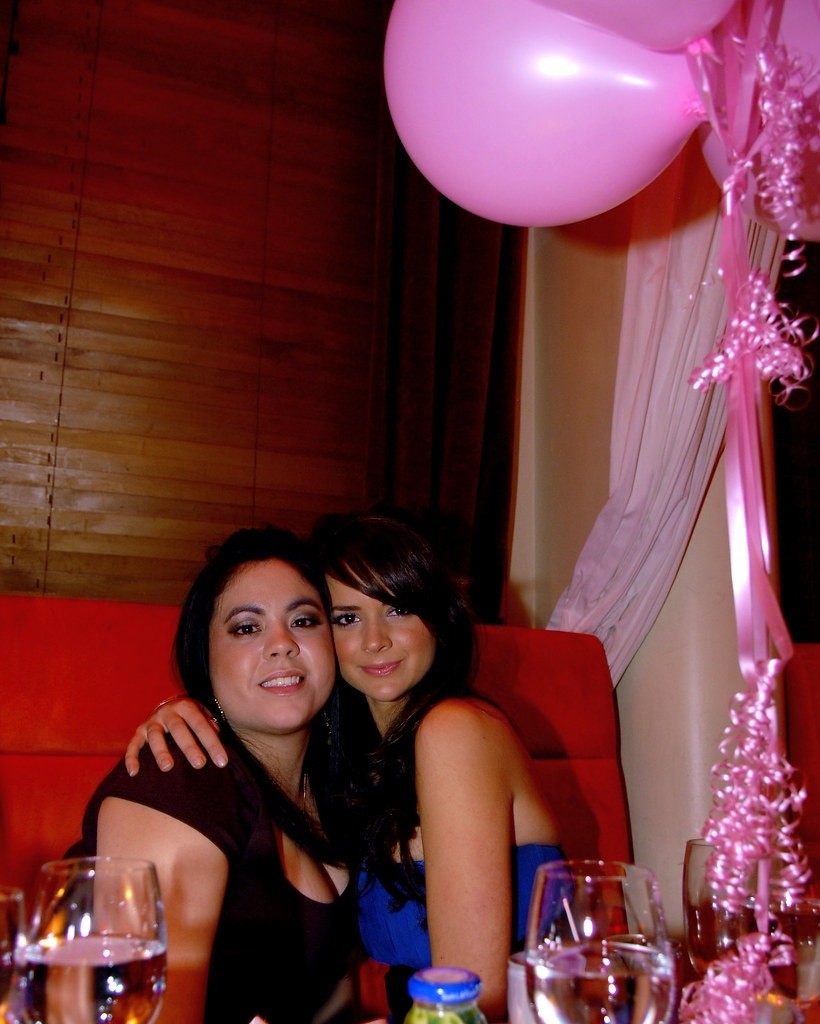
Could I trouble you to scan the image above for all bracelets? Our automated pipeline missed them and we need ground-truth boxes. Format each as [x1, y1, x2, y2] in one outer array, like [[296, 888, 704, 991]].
[[147, 698, 184, 716]]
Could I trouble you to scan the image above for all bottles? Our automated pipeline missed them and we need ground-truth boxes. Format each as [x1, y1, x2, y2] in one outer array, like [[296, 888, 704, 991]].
[[402, 965, 488, 1024]]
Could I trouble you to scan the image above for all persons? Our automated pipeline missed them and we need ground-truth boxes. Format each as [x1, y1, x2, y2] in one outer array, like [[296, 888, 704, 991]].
[[24, 524, 362, 1024], [125, 512, 628, 1024]]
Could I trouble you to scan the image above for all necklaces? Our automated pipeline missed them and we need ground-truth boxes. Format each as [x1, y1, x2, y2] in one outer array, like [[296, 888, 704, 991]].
[[303, 774, 306, 814]]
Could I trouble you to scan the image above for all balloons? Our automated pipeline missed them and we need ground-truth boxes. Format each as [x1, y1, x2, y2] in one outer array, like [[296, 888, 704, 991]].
[[546, 0, 737, 52], [384, 0, 704, 226], [699, 0, 820, 240]]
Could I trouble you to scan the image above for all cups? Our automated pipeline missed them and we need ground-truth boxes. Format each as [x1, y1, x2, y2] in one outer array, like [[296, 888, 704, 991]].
[[9, 856, 168, 1024], [505, 952, 537, 1024], [680, 838, 820, 1009], [522, 859, 677, 1024], [0, 885, 27, 1024]]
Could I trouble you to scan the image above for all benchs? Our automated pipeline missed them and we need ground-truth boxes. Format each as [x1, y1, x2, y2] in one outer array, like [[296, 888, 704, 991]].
[[0, 590, 633, 1024]]
